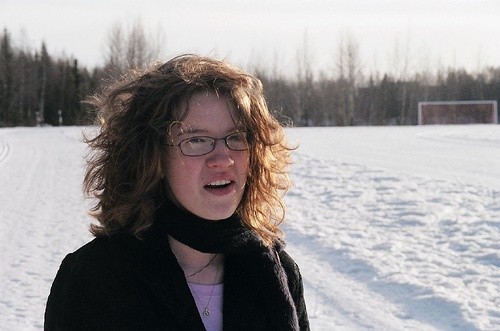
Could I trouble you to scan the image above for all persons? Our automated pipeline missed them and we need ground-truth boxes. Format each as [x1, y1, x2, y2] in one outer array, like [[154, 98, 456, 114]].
[[44, 53, 310, 331]]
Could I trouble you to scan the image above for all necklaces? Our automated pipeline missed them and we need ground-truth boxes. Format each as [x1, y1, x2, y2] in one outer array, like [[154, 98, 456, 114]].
[[184, 255, 221, 318]]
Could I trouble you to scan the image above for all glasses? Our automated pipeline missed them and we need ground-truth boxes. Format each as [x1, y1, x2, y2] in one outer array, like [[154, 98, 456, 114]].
[[160, 131, 256, 157]]
